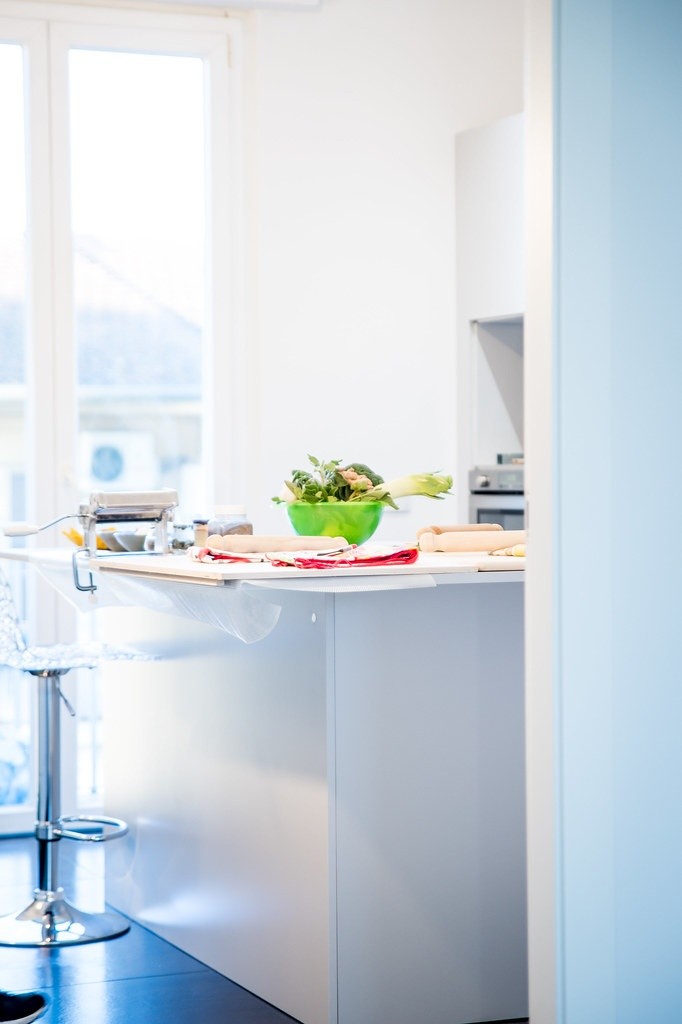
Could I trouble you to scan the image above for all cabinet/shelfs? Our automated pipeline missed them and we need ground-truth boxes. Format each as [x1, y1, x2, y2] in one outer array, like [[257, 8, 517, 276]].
[[470, 108, 523, 328]]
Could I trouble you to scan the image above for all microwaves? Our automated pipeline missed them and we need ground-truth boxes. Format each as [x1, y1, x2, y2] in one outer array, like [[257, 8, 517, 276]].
[[469, 465, 524, 531]]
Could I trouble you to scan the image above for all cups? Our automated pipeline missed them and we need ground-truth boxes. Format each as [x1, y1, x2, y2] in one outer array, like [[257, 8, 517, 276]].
[[193, 520, 208, 547]]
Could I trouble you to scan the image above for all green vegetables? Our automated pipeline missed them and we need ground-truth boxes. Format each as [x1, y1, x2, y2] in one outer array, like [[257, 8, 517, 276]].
[[272, 453, 399, 509]]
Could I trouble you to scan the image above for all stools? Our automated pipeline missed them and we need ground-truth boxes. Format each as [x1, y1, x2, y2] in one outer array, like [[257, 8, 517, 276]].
[[0, 514, 212, 954]]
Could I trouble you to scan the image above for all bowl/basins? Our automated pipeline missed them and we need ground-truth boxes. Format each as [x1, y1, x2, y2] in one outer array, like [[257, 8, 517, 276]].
[[114, 533, 147, 551], [99, 530, 135, 550], [288, 502, 381, 548]]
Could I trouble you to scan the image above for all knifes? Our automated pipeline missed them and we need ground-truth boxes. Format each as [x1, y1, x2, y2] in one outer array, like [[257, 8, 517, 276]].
[[317, 545, 352, 556]]
